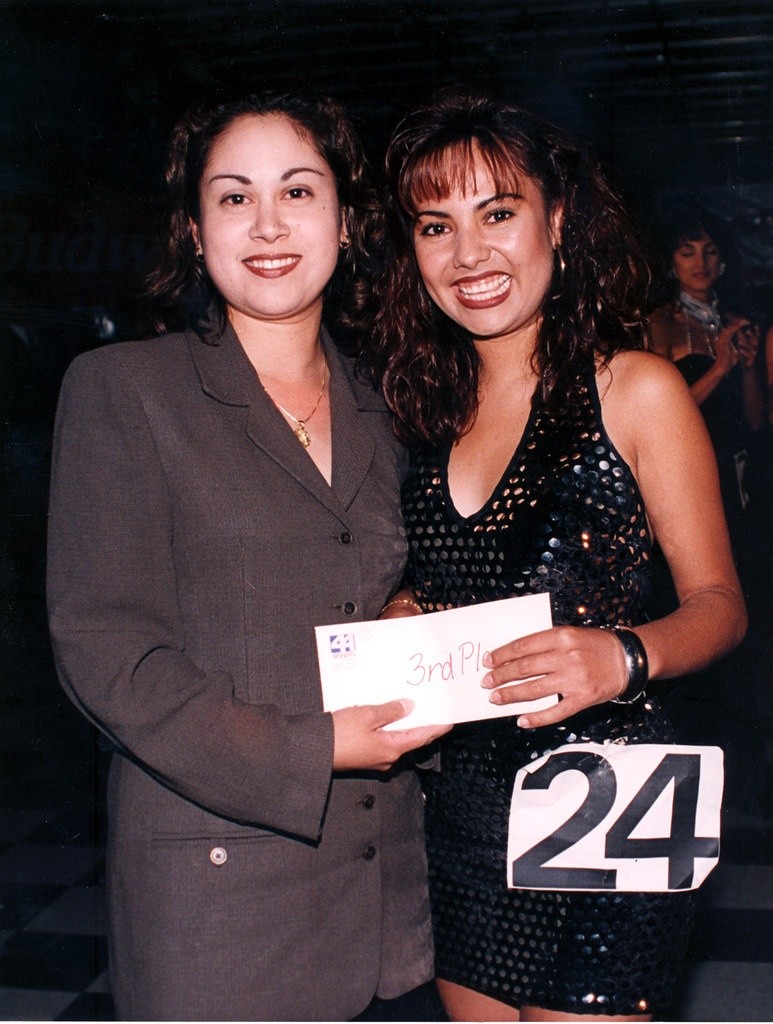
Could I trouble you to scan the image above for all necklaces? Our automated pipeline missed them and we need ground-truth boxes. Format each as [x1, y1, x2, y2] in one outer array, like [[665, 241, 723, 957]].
[[676, 288, 738, 360], [264, 340, 327, 447]]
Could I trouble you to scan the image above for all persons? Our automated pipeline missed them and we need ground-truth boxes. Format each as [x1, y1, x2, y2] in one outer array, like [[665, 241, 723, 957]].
[[45, 88, 452, 1022], [642, 208, 773, 817], [366, 94, 749, 1022]]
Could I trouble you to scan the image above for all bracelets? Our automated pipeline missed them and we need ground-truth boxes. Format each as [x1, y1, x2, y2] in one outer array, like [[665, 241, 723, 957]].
[[600, 624, 648, 703], [741, 360, 756, 372], [381, 599, 423, 613]]
